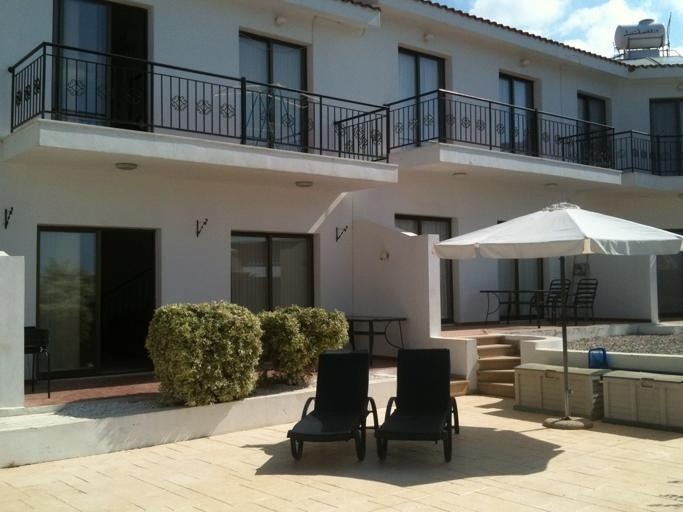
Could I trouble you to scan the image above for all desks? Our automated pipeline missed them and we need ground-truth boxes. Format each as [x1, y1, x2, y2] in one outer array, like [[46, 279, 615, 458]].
[[343, 317, 406, 369], [479, 289, 563, 329]]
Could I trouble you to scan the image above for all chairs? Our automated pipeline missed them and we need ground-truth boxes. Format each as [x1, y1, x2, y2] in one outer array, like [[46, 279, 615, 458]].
[[530, 279, 597, 326], [24, 326, 51, 399], [285, 348, 459, 462]]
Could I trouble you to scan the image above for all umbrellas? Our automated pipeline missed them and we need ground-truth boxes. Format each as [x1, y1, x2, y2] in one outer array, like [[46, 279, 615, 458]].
[[432, 202, 683, 430]]
